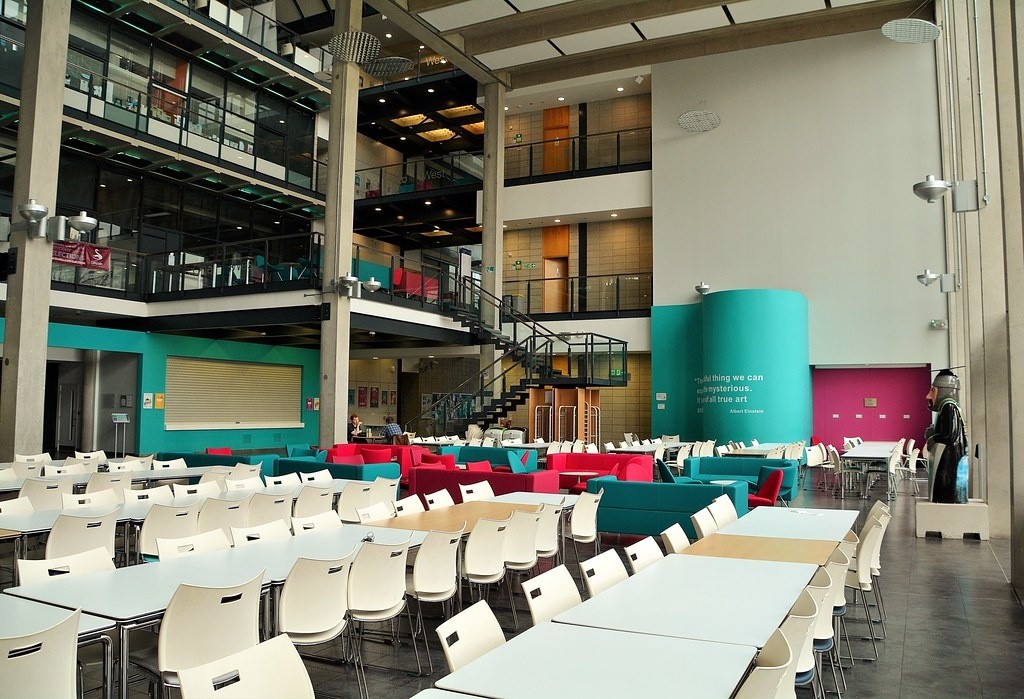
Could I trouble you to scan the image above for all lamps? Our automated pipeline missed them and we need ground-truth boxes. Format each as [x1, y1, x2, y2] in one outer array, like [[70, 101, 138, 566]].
[[16, 199, 49, 239], [913, 175, 979, 213], [352, 276, 381, 299], [917, 269, 955, 293], [47, 211, 98, 242], [337, 272, 357, 296], [694, 282, 710, 295]]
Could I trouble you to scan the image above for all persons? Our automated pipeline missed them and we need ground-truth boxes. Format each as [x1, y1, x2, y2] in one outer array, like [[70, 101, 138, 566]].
[[924, 369, 968, 504], [380, 417, 402, 444], [347, 414, 367, 443]]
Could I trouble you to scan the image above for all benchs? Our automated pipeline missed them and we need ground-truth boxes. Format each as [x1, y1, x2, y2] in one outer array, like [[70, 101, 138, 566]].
[[332, 443, 420, 484], [157, 452, 280, 487], [437, 446, 539, 471], [683, 457, 798, 507], [409, 465, 558, 511], [586, 475, 748, 544], [546, 453, 653, 490], [273, 457, 400, 502]]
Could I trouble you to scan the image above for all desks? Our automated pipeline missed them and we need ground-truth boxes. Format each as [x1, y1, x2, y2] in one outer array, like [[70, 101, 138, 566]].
[[162, 523, 429, 678], [0, 508, 132, 567], [840, 451, 892, 501], [2, 561, 271, 699], [478, 492, 581, 595], [229, 478, 373, 520], [610, 446, 660, 483], [677, 533, 840, 566], [352, 436, 387, 443], [36, 470, 151, 496], [135, 464, 233, 489], [97, 492, 253, 565], [503, 444, 547, 470], [550, 553, 819, 649], [358, 501, 541, 626], [558, 472, 598, 483], [721, 449, 770, 458], [434, 621, 757, 699], [715, 506, 861, 543]]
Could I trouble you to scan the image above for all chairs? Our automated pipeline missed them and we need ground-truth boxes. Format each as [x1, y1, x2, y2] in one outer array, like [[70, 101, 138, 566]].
[[65, 74, 253, 155], [0, 424, 929, 699]]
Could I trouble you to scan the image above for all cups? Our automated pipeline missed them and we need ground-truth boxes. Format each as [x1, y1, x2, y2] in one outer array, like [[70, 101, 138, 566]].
[[366, 429, 371, 438]]
